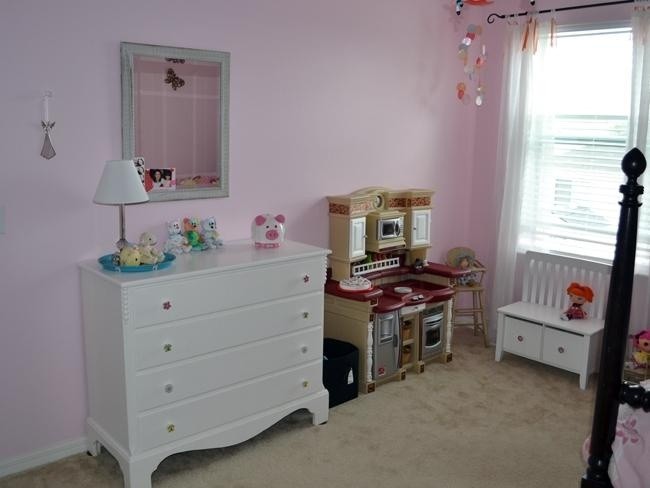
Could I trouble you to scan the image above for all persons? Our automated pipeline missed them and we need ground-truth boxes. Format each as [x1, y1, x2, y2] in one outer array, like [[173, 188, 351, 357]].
[[624, 330, 650, 369], [560, 282, 594, 321], [150, 170, 172, 187]]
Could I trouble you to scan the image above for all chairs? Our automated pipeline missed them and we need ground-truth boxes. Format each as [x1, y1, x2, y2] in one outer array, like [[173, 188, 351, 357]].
[[446, 256, 489, 348]]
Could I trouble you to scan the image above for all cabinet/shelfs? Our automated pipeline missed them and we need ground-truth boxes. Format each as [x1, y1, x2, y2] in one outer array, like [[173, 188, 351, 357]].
[[495, 300, 605, 391], [77, 238, 334, 488]]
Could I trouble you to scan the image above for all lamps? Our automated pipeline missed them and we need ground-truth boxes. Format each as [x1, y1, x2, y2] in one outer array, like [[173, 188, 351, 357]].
[[94, 160, 147, 249]]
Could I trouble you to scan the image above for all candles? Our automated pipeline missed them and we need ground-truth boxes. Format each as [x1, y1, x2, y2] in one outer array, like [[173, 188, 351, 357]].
[[42, 94, 50, 124]]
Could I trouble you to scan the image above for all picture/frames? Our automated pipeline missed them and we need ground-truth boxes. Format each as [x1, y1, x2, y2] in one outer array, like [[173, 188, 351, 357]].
[[121, 40, 231, 205]]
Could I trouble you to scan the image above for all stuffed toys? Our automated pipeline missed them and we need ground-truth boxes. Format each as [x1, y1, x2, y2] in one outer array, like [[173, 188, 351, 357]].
[[117, 216, 224, 266], [453, 256, 476, 287]]
